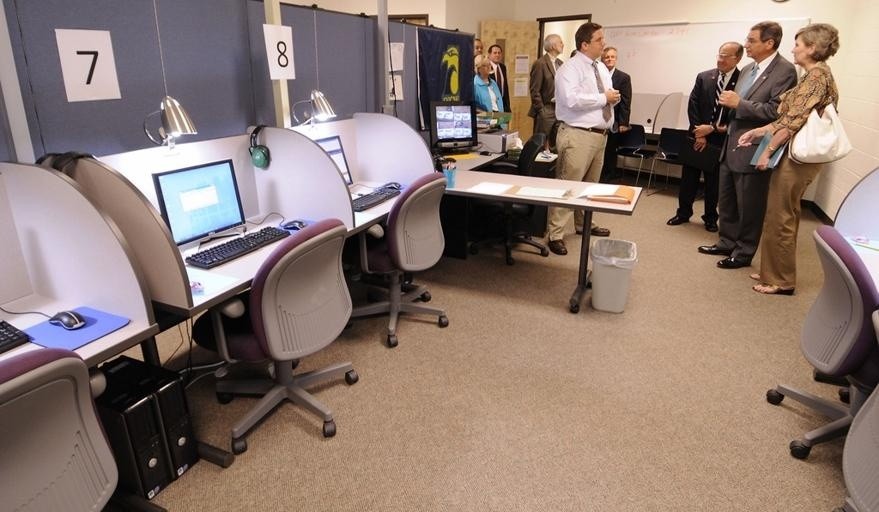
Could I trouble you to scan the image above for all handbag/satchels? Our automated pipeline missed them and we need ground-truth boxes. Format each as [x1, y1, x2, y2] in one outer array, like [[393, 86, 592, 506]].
[[787, 102, 853, 164]]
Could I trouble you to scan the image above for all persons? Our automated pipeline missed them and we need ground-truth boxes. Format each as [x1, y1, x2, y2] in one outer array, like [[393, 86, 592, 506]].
[[598, 47, 632, 184], [737, 24, 839, 295], [474, 54, 506, 130], [698, 21, 797, 269], [667, 42, 743, 232], [487, 44, 511, 112], [473, 38, 483, 57], [528, 34, 564, 153], [549, 22, 621, 254]]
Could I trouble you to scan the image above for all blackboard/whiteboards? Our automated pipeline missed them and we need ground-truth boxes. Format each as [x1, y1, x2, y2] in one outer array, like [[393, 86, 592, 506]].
[[601, 18, 812, 96]]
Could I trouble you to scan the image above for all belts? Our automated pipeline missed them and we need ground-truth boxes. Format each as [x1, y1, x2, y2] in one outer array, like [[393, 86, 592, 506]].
[[576, 125, 609, 134]]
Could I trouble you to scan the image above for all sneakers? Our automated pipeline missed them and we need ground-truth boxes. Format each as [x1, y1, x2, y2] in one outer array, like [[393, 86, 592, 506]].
[[750, 274, 794, 295]]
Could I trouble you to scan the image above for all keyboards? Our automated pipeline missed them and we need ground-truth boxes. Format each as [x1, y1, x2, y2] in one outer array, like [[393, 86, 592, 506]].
[[0, 320, 29, 354], [353, 189, 400, 212], [185, 226, 289, 269]]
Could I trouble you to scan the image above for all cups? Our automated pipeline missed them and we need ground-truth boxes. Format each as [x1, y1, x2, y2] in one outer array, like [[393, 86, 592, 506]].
[[442, 169, 457, 190]]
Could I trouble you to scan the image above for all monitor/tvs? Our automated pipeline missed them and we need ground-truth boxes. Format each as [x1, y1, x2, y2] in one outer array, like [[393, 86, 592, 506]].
[[313, 135, 353, 185], [429, 101, 477, 154], [152, 158, 246, 253]]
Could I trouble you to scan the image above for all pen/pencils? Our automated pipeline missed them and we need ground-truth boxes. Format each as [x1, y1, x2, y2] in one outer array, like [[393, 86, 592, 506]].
[[852, 242, 879, 251], [446, 161, 456, 170]]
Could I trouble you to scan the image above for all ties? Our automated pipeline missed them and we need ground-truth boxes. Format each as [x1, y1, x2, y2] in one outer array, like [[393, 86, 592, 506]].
[[708, 74, 726, 125], [593, 62, 611, 122], [496, 66, 502, 95], [737, 67, 758, 98]]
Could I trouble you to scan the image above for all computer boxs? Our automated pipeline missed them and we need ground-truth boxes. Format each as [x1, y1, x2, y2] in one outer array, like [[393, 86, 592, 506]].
[[98, 355, 202, 479], [89, 368, 176, 501]]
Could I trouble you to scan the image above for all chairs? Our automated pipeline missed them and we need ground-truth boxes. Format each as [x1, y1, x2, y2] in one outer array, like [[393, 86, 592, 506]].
[[463, 132, 549, 264], [831, 344, 879, 511], [216, 219, 359, 455], [613, 91, 691, 197], [347, 173, 449, 349], [766, 222, 879, 459], [1, 348, 125, 511]]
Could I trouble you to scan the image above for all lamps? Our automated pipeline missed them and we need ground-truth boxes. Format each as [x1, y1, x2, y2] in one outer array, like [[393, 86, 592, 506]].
[[291, 89, 338, 131], [140, 94, 197, 154]]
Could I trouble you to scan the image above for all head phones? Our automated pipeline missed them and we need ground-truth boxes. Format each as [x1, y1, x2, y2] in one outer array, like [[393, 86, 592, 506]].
[[248, 124, 270, 168]]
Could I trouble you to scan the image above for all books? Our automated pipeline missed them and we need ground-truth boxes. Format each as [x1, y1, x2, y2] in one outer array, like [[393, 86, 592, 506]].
[[578, 183, 635, 205]]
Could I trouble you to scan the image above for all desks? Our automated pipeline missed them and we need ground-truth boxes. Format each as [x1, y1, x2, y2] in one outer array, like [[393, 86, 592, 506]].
[[2, 159, 165, 510], [290, 112, 434, 311], [813, 165, 878, 388], [446, 168, 645, 314], [60, 124, 354, 471], [422, 128, 559, 258]]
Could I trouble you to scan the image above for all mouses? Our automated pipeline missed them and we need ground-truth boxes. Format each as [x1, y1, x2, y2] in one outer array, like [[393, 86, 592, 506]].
[[284, 220, 304, 230], [385, 182, 399, 189], [480, 151, 491, 156], [49, 311, 85, 330]]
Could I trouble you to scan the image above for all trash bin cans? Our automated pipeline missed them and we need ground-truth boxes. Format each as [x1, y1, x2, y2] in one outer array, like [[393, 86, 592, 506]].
[[589, 238, 637, 313]]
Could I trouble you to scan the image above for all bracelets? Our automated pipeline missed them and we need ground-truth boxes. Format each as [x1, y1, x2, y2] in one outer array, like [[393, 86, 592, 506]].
[[712, 123, 718, 134], [768, 146, 777, 152]]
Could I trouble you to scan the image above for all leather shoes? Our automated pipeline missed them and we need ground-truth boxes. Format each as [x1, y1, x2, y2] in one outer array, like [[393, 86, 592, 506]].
[[667, 217, 690, 225], [705, 220, 718, 230], [576, 227, 610, 236], [718, 258, 748, 269], [548, 238, 567, 255], [699, 246, 732, 254]]
